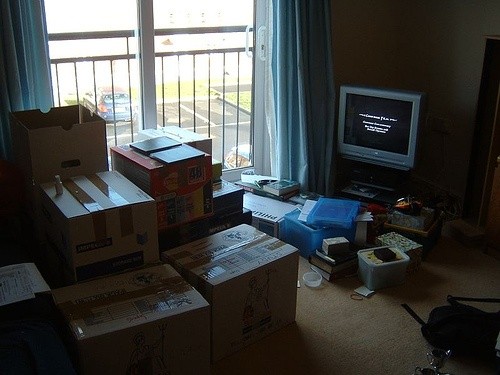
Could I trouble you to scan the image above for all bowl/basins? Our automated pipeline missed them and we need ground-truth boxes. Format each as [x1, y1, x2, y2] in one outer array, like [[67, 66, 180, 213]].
[[303, 272, 322, 287]]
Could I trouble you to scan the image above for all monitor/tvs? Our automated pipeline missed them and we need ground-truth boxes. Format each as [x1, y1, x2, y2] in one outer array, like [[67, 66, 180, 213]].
[[338, 83, 426, 172]]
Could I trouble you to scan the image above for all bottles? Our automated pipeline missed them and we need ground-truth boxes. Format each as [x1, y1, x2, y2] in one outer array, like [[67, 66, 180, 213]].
[[54, 174, 63, 195]]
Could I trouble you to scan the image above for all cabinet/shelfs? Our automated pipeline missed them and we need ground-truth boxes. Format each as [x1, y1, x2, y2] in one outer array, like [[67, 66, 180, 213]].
[[336, 177, 437, 206]]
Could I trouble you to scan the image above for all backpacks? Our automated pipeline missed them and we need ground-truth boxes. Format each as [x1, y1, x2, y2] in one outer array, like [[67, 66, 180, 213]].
[[401, 295, 500, 360]]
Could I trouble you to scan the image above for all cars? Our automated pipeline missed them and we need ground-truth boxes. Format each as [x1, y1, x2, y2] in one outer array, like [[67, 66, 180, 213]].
[[98, 93, 136, 123]]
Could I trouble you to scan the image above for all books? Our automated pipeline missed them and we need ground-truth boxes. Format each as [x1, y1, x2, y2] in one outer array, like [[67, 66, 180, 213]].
[[264, 179, 301, 197], [309, 237, 372, 282]]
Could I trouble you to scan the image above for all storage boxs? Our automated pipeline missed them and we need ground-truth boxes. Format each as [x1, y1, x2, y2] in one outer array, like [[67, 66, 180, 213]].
[[7, 105, 443, 375]]
